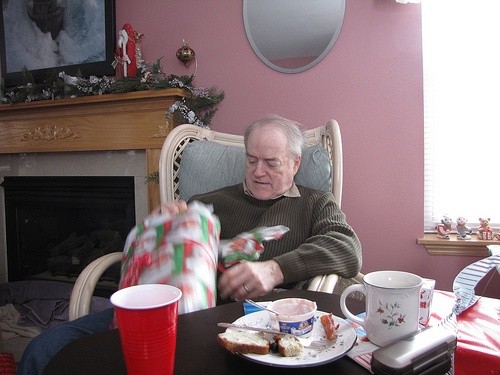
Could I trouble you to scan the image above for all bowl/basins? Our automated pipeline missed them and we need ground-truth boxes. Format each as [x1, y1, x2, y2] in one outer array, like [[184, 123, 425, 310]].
[[267, 298, 317, 337]]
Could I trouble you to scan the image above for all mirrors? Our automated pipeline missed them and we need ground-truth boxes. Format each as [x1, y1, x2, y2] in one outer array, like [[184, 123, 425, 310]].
[[243, 1, 345, 75]]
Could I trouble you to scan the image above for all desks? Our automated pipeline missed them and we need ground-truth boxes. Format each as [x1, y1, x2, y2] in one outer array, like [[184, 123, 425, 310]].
[[0, 88, 205, 290]]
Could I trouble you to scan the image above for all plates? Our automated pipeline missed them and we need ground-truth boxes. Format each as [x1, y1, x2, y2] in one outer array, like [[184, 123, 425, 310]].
[[224, 309, 357, 368]]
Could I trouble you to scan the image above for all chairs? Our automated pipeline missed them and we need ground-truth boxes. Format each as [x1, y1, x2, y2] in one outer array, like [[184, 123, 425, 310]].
[[161, 119, 343, 294]]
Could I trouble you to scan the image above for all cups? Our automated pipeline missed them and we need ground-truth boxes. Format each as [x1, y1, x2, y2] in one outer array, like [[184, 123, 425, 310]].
[[340, 270, 424, 348], [110, 283, 182, 375]]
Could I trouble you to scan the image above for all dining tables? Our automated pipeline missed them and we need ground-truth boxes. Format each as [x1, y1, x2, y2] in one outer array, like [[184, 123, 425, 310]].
[[41, 289, 452, 375]]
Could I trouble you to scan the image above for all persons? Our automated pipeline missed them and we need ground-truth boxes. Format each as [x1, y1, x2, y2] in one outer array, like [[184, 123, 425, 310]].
[[18, 115, 362, 375]]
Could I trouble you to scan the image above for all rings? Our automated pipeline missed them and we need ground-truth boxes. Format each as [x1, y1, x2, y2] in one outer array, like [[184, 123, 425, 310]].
[[243, 284, 249, 292]]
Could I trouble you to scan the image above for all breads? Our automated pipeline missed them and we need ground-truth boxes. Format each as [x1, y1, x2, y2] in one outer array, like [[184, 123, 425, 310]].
[[217, 331, 301, 357]]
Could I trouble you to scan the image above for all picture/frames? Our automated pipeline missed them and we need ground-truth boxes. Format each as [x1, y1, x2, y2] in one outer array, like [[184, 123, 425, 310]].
[[0, 0, 116, 91]]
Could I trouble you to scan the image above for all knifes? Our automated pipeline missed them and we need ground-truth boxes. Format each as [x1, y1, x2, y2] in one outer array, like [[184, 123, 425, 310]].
[[218, 322, 286, 335]]
